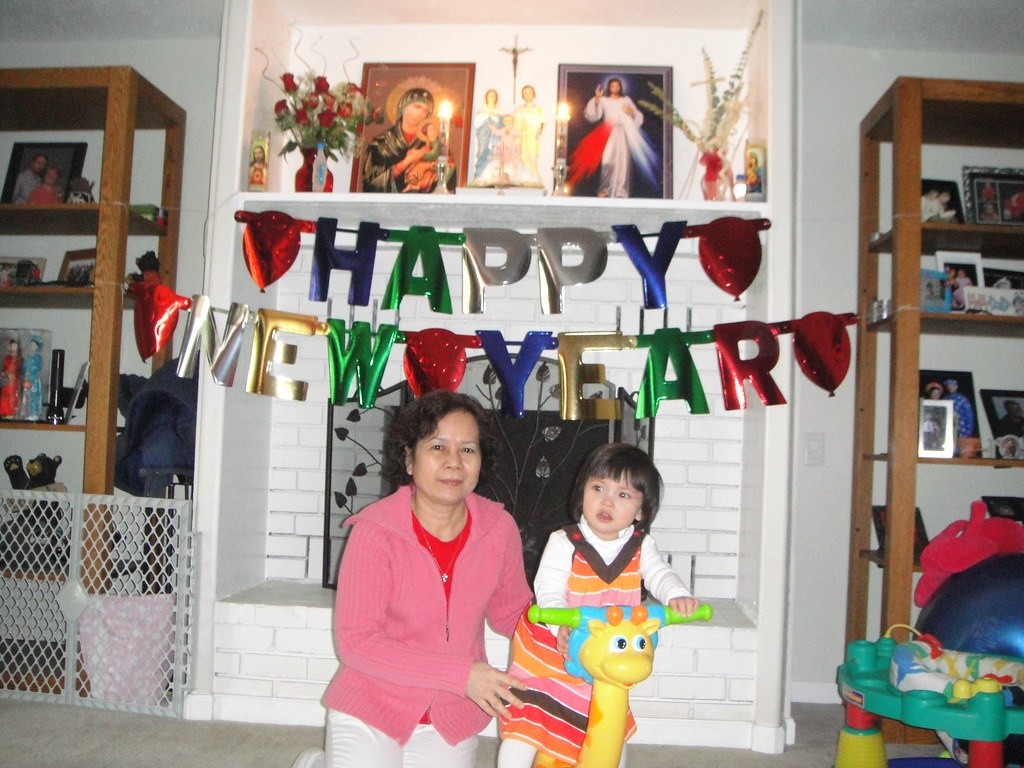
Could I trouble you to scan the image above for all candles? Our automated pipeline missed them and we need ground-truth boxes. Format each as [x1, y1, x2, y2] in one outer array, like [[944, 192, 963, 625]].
[[436, 100, 454, 157], [554, 104, 568, 161]]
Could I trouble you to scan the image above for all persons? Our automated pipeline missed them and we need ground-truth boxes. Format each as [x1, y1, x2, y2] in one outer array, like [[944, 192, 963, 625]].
[[495, 442, 700, 768], [920, 184, 1024, 309], [921, 379, 975, 453], [21, 335, 45, 420], [999, 400, 1024, 437], [12, 152, 48, 206], [0, 340, 23, 422], [319, 391, 534, 768], [28, 164, 61, 205]]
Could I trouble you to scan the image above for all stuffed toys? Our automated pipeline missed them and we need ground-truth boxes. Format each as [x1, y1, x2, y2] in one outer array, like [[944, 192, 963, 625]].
[[4, 453, 63, 528], [914, 500, 1024, 607]]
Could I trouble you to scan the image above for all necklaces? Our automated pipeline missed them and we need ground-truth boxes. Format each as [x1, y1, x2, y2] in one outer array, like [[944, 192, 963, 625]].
[[414, 493, 461, 583]]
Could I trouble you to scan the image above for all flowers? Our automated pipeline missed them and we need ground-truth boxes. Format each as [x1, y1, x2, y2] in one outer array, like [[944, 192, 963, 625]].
[[641, 11, 764, 163], [273, 70, 373, 152]]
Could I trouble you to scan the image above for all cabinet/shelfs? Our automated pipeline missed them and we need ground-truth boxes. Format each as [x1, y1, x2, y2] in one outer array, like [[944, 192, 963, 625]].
[[0, 65, 187, 693], [838, 73, 1023, 742]]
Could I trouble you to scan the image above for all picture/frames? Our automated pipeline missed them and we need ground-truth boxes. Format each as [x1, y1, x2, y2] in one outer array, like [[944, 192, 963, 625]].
[[350, 62, 478, 193], [935, 249, 985, 311], [0, 256, 46, 286], [981, 494, 1023, 525], [57, 246, 99, 287], [1, 141, 88, 203], [874, 508, 929, 559], [920, 271, 951, 311], [554, 63, 676, 195], [917, 397, 954, 457], [980, 389, 1023, 468], [962, 166, 1023, 225]]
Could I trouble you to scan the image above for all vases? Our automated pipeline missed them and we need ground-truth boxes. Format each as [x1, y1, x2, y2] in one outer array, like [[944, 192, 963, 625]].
[[295, 139, 334, 194], [700, 151, 733, 201]]
